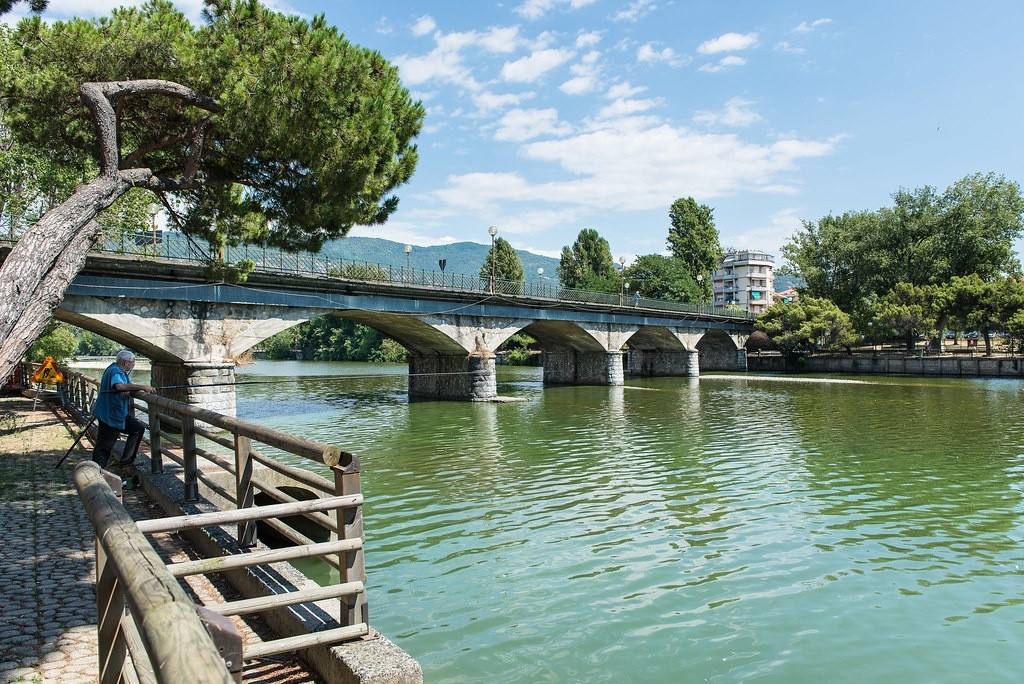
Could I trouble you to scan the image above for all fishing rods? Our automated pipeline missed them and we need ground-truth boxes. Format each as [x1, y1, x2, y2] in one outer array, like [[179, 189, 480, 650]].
[[100, 377, 343, 393]]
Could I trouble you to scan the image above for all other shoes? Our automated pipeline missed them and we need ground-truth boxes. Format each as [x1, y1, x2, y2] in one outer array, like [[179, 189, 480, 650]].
[[121, 460, 144, 468]]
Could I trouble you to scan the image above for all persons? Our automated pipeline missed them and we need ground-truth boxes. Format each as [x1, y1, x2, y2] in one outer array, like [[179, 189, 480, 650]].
[[632, 291, 641, 308], [92, 349, 156, 478]]
